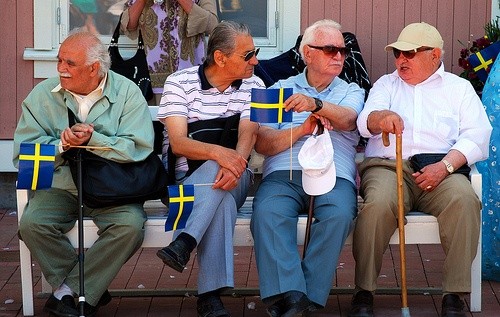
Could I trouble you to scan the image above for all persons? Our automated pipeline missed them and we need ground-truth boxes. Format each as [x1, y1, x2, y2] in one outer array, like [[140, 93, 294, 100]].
[[350, 22, 493, 317], [250, 18, 365, 317], [156, 20, 266, 317], [11, 31, 155, 316], [117, 0, 219, 156]]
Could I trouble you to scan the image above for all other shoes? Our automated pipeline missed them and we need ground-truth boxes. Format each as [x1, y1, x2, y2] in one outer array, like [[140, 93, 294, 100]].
[[76, 289, 113, 317], [43, 295, 80, 317]]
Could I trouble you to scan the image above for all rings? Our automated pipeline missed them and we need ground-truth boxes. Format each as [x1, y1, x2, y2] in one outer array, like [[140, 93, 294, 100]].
[[426, 185, 432, 190]]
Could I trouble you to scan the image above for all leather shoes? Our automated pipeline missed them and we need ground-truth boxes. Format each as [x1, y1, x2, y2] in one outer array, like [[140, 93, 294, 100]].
[[280, 292, 318, 317], [265, 299, 290, 317]]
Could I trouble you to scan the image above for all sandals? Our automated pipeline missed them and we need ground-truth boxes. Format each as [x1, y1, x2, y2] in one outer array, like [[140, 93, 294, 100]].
[[195, 298, 231, 317], [156, 240, 189, 273]]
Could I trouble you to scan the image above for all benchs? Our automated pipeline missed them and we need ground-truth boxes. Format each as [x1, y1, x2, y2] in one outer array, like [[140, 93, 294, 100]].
[[14, 106, 482, 317]]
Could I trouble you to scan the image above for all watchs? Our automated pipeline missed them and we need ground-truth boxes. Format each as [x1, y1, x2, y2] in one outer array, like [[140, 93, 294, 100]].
[[311, 97, 323, 113], [441, 159, 454, 174]]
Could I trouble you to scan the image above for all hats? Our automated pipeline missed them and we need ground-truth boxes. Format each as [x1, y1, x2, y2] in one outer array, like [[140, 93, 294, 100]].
[[384, 22, 443, 51], [298, 124, 336, 195]]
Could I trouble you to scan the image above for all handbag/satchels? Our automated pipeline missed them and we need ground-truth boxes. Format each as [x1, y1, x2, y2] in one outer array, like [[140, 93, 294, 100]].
[[106, 12, 153, 102], [187, 113, 250, 175], [69, 146, 171, 208], [410, 152, 472, 179]]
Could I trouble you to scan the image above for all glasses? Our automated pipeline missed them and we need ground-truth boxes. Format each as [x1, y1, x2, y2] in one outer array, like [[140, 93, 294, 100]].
[[392, 47, 433, 59], [308, 45, 351, 59], [222, 47, 260, 61]]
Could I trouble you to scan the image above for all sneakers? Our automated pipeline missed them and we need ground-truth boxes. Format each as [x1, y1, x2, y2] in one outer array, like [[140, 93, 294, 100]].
[[441, 294, 467, 317], [350, 290, 373, 317]]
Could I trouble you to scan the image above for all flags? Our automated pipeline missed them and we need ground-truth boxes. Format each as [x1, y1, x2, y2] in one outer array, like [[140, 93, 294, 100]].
[[164, 184, 195, 232], [17, 142, 56, 191], [251, 89, 292, 123]]
